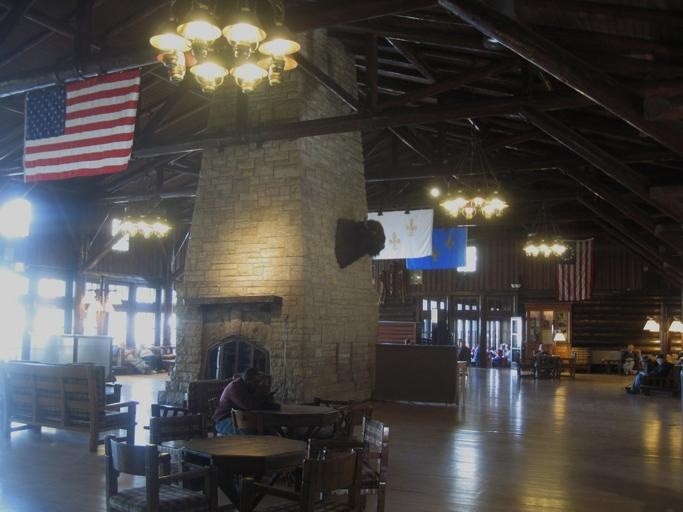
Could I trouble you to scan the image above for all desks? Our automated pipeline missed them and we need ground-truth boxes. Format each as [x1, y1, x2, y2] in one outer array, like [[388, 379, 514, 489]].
[[236, 403, 340, 493], [187, 433, 307, 512]]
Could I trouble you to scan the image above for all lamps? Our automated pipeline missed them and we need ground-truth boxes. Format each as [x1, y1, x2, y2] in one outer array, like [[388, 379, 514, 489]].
[[429, 130, 514, 222], [111, 172, 171, 239], [554, 329, 567, 354], [522, 200, 571, 260], [148, 1, 302, 95]]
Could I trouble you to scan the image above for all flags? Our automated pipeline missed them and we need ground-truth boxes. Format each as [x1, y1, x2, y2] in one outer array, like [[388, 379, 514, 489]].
[[23, 68, 142, 181], [405, 226, 468, 270], [556, 239, 593, 302], [366, 208, 435, 260]]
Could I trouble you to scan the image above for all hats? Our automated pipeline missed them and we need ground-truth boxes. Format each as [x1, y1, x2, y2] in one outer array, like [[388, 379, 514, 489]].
[[655, 354, 664, 359], [639, 355, 650, 362]]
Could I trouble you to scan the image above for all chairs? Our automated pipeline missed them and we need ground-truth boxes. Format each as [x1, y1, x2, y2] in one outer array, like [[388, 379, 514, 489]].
[[230, 408, 263, 436], [145, 348, 157, 370], [302, 416, 389, 512], [253, 449, 361, 512], [207, 397, 220, 438], [333, 400, 354, 440], [623, 350, 642, 375], [296, 396, 347, 437], [111, 349, 122, 373], [640, 365, 682, 395], [515, 352, 535, 377], [105, 435, 209, 512], [560, 351, 576, 379], [157, 347, 170, 371], [123, 348, 139, 374], [536, 354, 560, 380], [150, 414, 209, 490]]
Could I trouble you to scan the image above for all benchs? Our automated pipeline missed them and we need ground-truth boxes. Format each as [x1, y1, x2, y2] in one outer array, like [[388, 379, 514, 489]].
[[590, 349, 622, 374]]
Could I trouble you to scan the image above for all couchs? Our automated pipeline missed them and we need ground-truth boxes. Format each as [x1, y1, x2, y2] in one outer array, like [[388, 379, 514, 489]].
[[0, 360, 139, 453], [144, 378, 235, 431]]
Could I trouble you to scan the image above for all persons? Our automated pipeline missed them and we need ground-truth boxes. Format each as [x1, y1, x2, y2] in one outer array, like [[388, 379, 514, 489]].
[[532, 343, 552, 377], [212, 368, 282, 441], [620, 344, 639, 376], [655, 354, 671, 375], [678, 352, 683, 366], [116, 339, 164, 375], [625, 355, 658, 393], [455, 333, 511, 367], [403, 337, 416, 347]]
